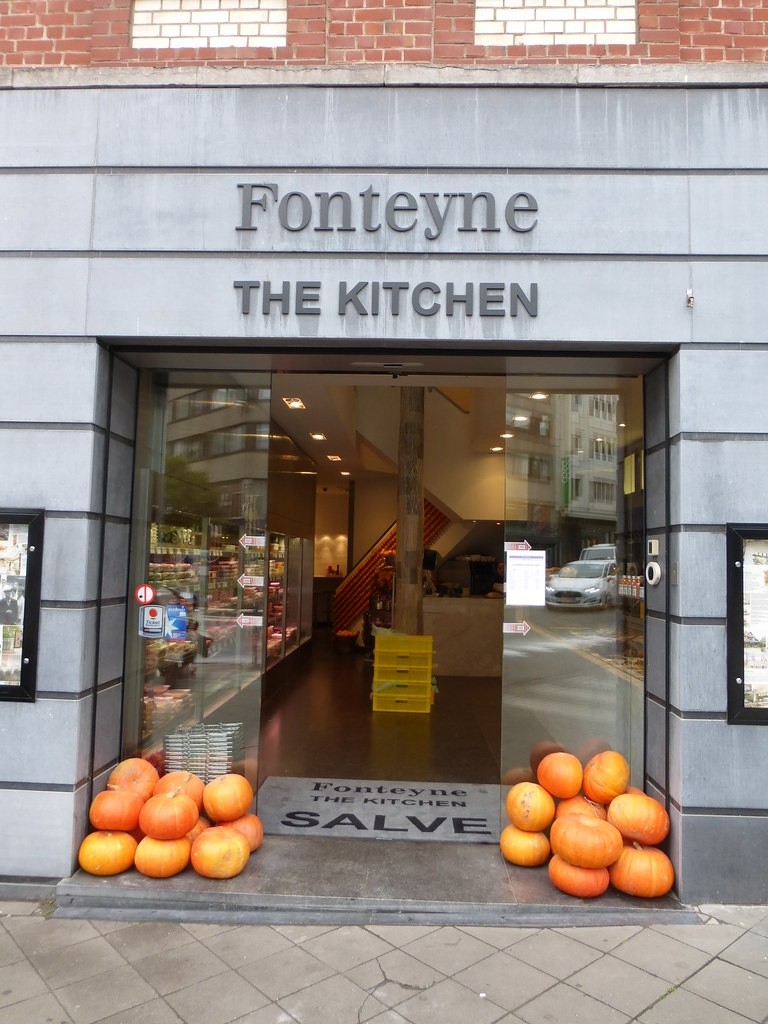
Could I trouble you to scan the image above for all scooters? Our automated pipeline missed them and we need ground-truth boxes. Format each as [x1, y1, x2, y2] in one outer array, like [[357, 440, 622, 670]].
[[161, 584, 212, 672]]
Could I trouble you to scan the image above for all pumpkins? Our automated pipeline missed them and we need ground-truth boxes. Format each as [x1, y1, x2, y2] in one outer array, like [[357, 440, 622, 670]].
[[79, 757, 263, 879], [500, 752, 674, 898]]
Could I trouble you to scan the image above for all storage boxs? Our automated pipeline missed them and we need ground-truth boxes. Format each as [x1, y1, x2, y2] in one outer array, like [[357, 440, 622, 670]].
[[371, 633, 433, 712]]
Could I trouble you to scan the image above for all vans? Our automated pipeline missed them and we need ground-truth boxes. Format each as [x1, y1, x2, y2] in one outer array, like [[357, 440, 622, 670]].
[[580, 544, 618, 567]]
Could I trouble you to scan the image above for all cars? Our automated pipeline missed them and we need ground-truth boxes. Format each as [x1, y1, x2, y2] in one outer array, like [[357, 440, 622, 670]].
[[545, 562, 620, 609]]
